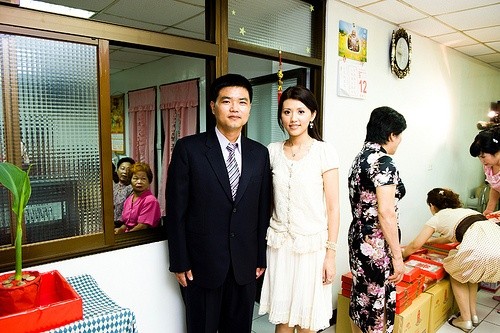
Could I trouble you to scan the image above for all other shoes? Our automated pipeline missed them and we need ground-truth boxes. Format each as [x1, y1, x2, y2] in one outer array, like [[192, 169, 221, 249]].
[[492, 296, 500, 300], [497, 309, 500, 313]]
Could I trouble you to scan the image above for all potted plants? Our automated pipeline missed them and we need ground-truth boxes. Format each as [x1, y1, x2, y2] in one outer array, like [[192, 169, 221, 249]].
[[0, 162, 41, 314]]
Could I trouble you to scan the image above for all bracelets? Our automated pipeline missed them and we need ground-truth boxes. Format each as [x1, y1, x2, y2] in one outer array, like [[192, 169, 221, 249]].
[[325, 241, 336, 251]]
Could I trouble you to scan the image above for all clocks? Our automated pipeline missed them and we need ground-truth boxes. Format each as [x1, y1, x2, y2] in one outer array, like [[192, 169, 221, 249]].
[[396, 37, 409, 70]]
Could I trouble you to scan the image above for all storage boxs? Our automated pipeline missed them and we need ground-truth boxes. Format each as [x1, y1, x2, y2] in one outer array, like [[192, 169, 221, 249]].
[[425, 278, 454, 333], [336, 291, 432, 333], [1, 270, 83, 333]]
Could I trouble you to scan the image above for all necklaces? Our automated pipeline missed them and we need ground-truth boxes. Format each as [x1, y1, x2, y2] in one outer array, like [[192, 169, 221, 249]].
[[290, 138, 314, 157]]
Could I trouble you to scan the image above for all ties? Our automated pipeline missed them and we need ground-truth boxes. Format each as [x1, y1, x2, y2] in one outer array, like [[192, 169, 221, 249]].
[[225, 143, 241, 201]]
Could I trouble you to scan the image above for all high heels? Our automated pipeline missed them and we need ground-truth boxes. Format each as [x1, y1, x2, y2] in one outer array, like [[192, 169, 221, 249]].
[[456, 311, 479, 327], [447, 316, 472, 333]]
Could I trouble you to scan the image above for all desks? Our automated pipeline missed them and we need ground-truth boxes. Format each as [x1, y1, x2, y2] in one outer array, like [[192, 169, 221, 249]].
[[41, 274, 138, 333]]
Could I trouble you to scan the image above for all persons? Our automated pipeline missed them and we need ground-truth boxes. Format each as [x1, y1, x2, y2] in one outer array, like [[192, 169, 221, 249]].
[[266, 85, 340, 333], [112, 163, 117, 186], [114, 158, 135, 228], [470, 124, 500, 300], [347, 106, 407, 333], [165, 73, 274, 333], [114, 162, 161, 233], [400, 187, 500, 333]]
